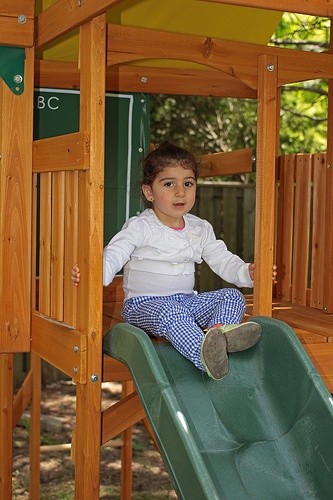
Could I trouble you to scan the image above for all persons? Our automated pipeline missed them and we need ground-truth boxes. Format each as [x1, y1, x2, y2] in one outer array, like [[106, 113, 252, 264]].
[[71, 141, 277, 380]]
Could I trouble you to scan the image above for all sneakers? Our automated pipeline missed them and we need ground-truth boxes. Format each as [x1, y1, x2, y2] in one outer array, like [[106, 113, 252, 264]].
[[200, 328, 229, 381], [203, 322, 262, 352]]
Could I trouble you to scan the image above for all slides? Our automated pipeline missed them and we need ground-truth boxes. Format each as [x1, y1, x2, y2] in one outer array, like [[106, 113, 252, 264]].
[[104, 315, 333, 496]]
